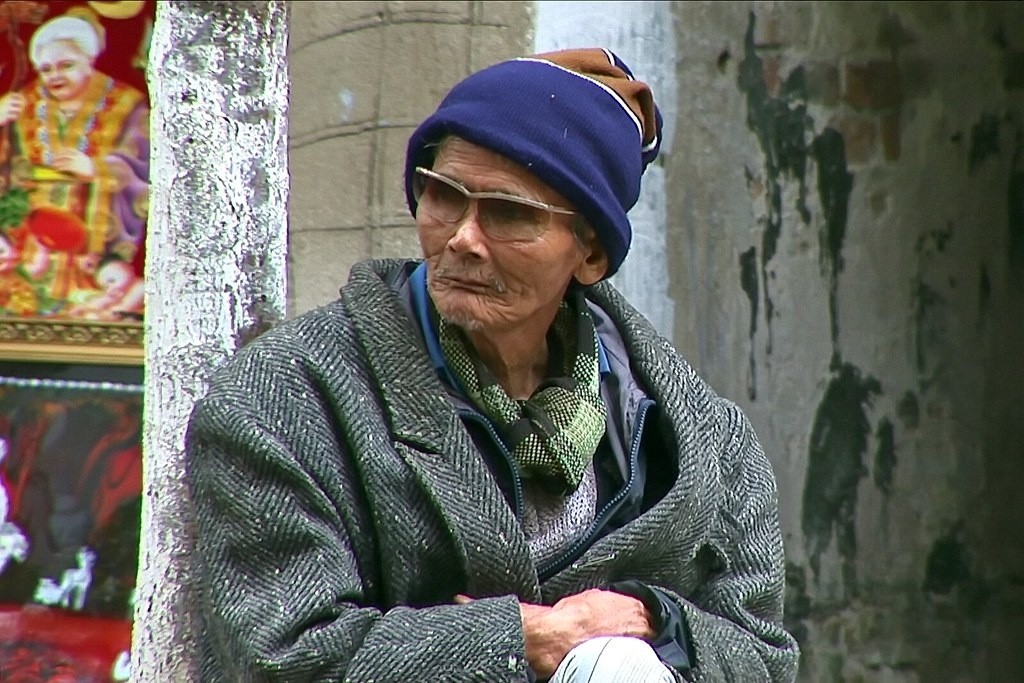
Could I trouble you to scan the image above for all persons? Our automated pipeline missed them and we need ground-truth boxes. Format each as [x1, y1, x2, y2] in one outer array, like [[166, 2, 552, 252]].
[[185, 49, 799, 683]]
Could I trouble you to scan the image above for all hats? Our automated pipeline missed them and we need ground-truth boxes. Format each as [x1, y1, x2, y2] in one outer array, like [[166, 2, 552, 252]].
[[405, 45, 663, 281]]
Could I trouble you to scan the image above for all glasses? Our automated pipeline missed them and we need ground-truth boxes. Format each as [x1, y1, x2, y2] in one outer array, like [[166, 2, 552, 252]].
[[412, 165, 577, 242]]
